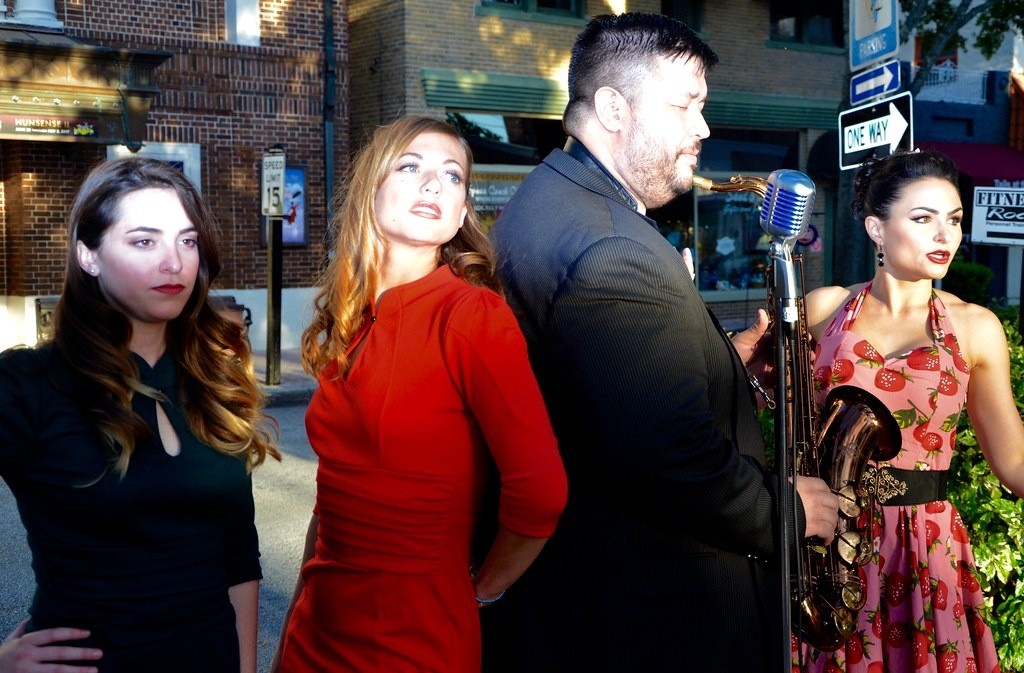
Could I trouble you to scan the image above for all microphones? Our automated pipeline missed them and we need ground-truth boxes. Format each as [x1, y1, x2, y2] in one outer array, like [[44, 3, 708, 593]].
[[759, 170, 816, 341]]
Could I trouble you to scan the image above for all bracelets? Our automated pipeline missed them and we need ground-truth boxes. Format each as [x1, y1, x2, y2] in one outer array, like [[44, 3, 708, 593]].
[[475, 590, 505, 607]]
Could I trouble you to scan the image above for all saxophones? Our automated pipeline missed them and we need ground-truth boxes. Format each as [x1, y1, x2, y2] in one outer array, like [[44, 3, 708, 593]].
[[689, 173, 904, 652]]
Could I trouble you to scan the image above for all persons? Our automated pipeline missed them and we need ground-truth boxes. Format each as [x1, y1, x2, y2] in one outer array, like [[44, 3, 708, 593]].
[[469, 13, 840, 673], [0, 158, 283, 673], [799, 155, 1022, 673], [270, 116, 568, 672]]
